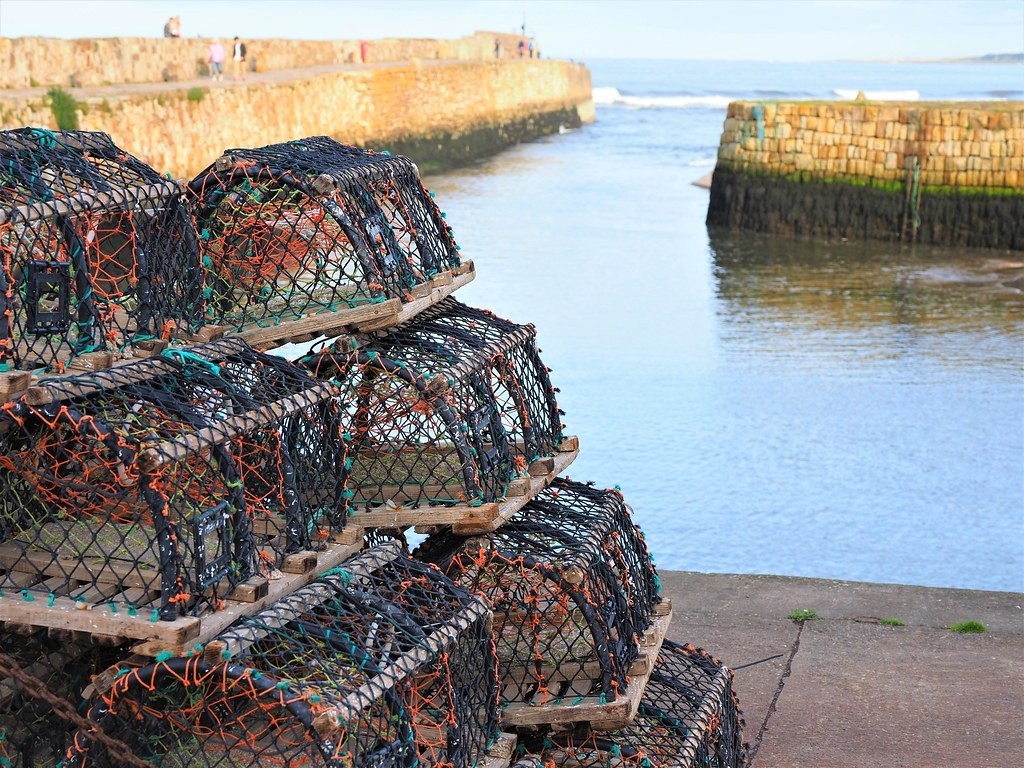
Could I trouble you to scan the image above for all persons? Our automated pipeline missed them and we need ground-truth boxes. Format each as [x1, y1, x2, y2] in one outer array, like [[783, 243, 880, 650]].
[[493, 39, 534, 59], [164, 16, 180, 38], [232, 36, 247, 81], [208, 38, 224, 81]]
